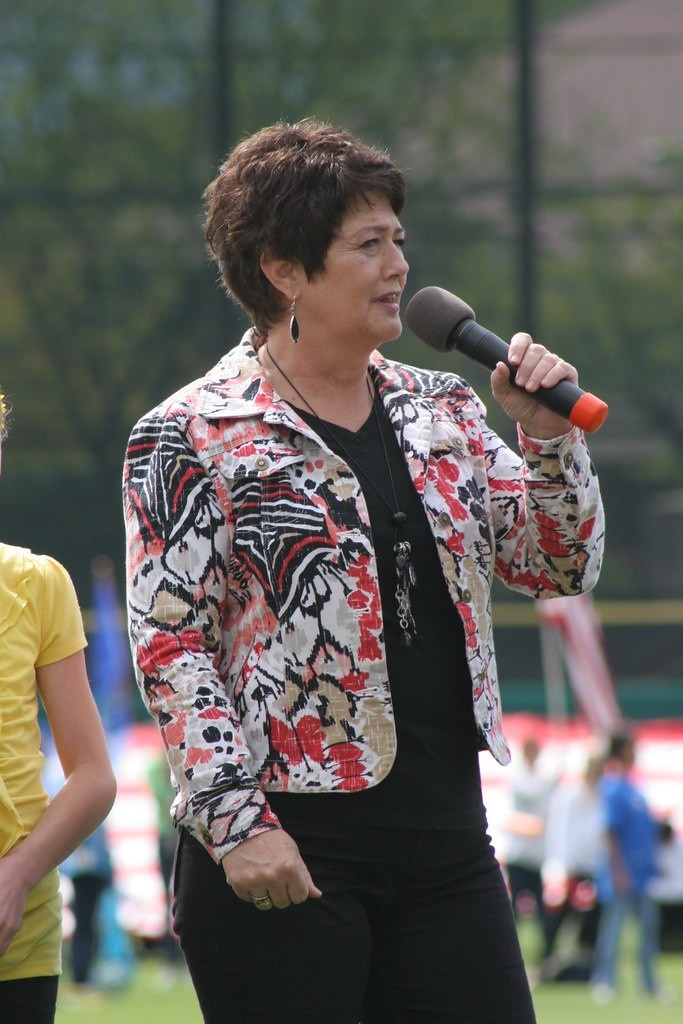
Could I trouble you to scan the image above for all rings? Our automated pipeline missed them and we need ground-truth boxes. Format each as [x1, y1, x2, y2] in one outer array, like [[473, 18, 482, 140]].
[[252, 896, 272, 908]]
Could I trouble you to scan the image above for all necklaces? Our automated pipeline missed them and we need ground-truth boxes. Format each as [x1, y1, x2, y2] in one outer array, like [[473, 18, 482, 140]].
[[260, 335, 420, 647]]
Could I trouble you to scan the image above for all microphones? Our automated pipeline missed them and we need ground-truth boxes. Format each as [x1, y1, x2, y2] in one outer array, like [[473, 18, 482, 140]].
[[405, 286, 608, 433]]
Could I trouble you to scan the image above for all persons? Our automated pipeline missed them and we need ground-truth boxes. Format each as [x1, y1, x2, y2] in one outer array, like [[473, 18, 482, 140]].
[[501, 728, 667, 990], [122, 116, 604, 1024], [0, 542, 183, 1024]]
